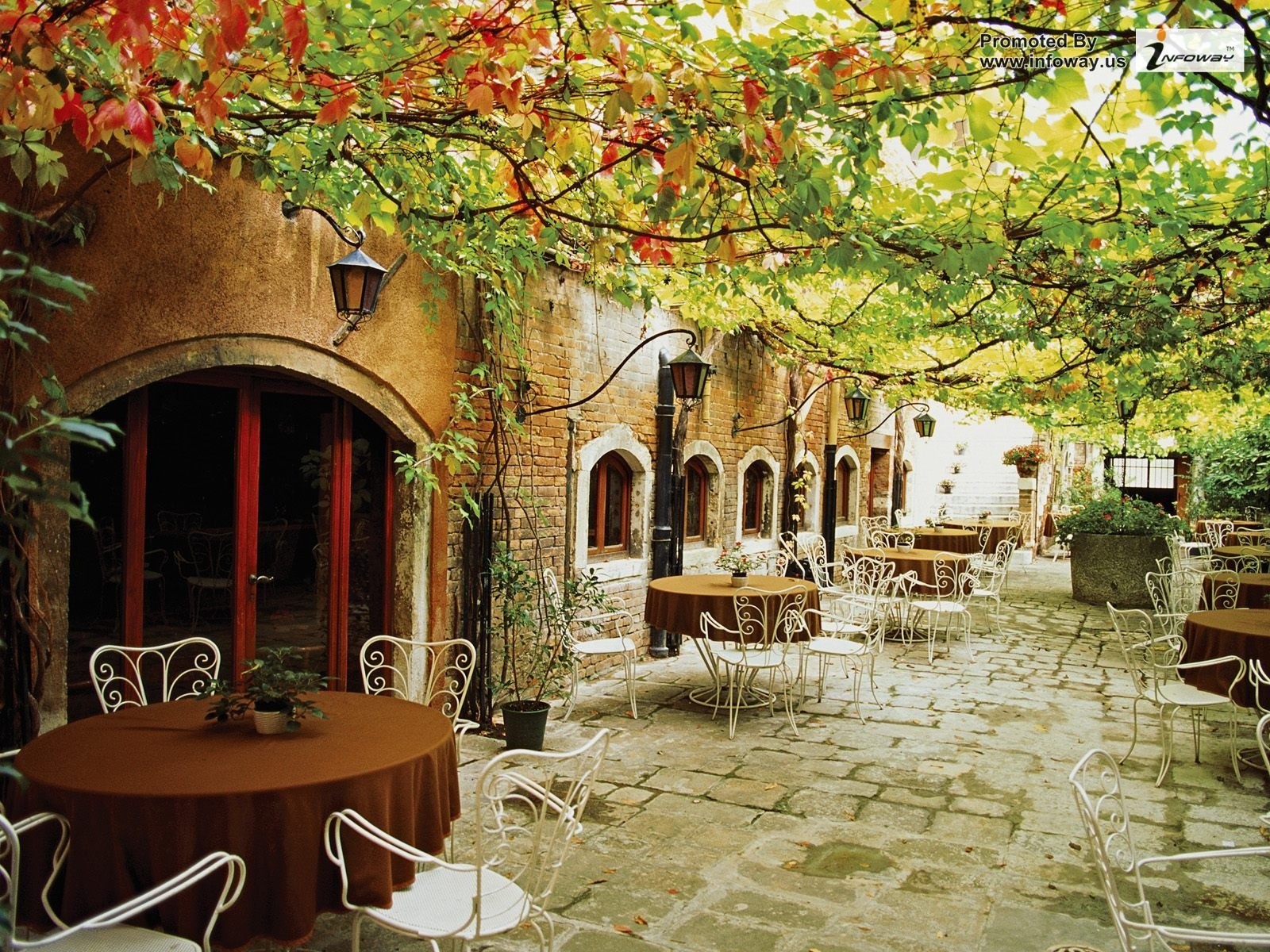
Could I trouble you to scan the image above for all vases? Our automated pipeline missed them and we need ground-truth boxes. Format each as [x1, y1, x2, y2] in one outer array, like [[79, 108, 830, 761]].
[[959, 448, 964, 455], [940, 510, 945, 516], [944, 485, 951, 494], [977, 515, 989, 521], [729, 575, 746, 585], [934, 524, 942, 532], [1016, 460, 1038, 477], [897, 543, 911, 553], [954, 466, 960, 473], [1071, 533, 1171, 613]]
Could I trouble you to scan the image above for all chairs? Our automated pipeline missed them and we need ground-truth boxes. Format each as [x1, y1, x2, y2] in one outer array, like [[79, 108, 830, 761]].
[[1064, 512, 1270, 951], [0, 511, 1034, 952]]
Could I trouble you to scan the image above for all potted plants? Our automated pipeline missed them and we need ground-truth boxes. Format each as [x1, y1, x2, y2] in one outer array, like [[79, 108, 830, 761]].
[[208, 642, 325, 748], [487, 544, 607, 753]]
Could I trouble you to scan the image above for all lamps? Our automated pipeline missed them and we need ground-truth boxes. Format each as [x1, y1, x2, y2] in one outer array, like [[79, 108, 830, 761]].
[[284, 195, 386, 333], [844, 399, 936, 440], [733, 375, 870, 436], [514, 323, 712, 425]]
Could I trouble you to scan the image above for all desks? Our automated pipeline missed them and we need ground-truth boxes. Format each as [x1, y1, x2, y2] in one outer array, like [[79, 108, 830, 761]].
[[1211, 545, 1270, 572], [935, 520, 1023, 580], [643, 575, 819, 711], [1213, 521, 1257, 543], [1193, 518, 1230, 539], [19, 689, 462, 952], [1224, 530, 1269, 546], [884, 527, 981, 554], [1176, 609, 1270, 769], [841, 547, 969, 644], [1197, 571, 1269, 611]]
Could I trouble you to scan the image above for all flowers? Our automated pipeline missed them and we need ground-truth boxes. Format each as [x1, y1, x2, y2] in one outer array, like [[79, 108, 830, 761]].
[[957, 443, 968, 449], [1003, 445, 1047, 464], [1052, 483, 1189, 539], [953, 462, 965, 468], [980, 510, 990, 517], [939, 478, 957, 487], [897, 526, 919, 546], [936, 504, 949, 513], [714, 539, 768, 571], [933, 519, 945, 528]]
